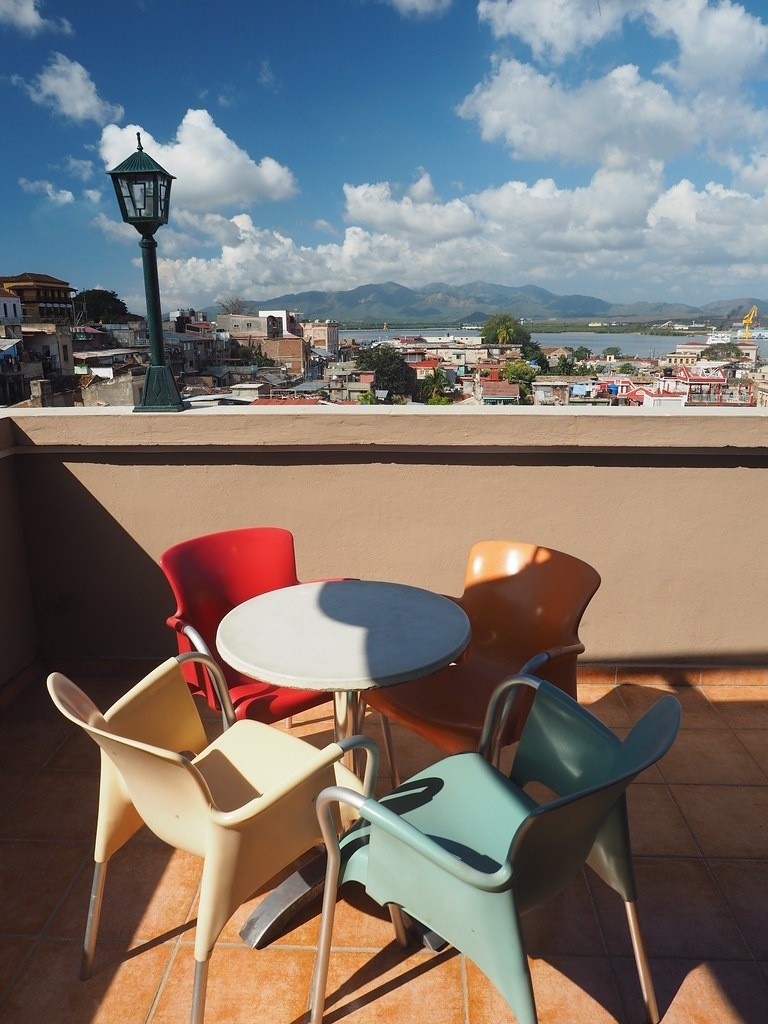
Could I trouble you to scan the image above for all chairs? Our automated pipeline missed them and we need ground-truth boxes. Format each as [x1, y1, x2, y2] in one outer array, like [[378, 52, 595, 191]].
[[43, 525, 681, 1024]]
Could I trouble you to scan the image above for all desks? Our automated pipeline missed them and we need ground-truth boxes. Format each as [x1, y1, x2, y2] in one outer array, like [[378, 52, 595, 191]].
[[215, 580, 471, 950]]
